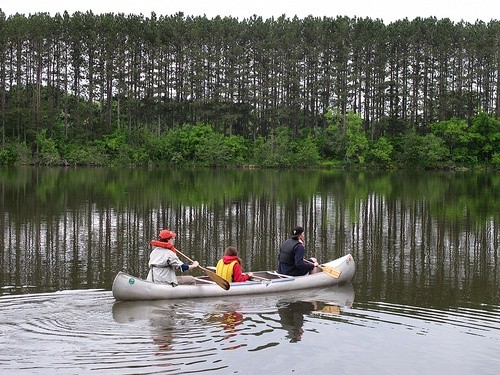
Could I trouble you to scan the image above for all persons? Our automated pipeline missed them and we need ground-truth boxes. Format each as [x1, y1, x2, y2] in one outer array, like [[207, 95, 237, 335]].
[[216, 247, 253, 283], [154, 336, 173, 354], [146, 230, 199, 285], [277, 301, 324, 343], [210, 311, 243, 333], [277, 226, 319, 277]]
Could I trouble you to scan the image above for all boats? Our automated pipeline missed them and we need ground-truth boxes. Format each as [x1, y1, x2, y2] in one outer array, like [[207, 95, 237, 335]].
[[111, 253, 355, 301]]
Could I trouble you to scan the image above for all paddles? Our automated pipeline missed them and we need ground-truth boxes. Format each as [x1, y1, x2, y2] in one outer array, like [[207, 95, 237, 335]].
[[303, 256, 341, 279], [176, 248, 230, 292], [204, 264, 270, 283], [316, 302, 341, 317]]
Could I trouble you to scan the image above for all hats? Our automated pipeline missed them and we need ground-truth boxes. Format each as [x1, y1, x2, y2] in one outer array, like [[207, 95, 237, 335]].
[[291, 226, 304, 238], [158, 230, 176, 239]]
